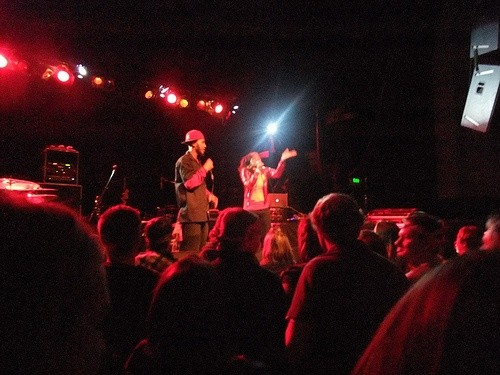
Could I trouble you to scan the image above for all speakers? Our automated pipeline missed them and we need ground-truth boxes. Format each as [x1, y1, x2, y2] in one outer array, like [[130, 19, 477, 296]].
[[459, 16, 500, 132], [38, 182, 83, 215]]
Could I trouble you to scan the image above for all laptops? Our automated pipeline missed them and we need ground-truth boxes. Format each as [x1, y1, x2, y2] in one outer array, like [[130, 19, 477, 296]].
[[267, 193, 289, 207]]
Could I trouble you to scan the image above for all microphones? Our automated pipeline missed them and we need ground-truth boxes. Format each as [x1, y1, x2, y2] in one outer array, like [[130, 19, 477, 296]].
[[210, 169, 214, 180], [256, 161, 260, 169]]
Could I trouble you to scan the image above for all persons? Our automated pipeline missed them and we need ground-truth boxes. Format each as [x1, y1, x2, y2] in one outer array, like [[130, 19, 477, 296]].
[[240, 148, 297, 244], [99, 205, 159, 375], [175, 130, 217, 253], [136, 193, 500, 375], [0, 197, 108, 375], [395, 212, 447, 282]]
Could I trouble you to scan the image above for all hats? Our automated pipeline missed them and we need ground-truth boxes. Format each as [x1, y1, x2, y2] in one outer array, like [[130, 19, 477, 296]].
[[181, 129, 204, 144]]
